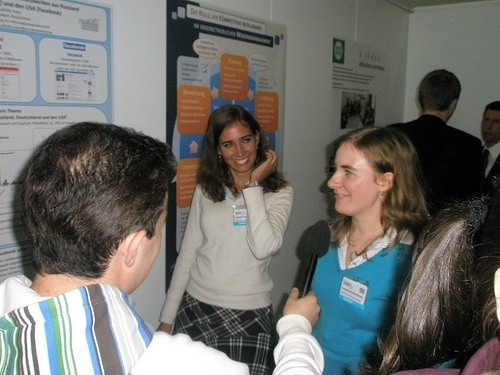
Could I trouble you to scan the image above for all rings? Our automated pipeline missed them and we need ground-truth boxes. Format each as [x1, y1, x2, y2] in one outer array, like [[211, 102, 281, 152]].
[[272, 159, 276, 162]]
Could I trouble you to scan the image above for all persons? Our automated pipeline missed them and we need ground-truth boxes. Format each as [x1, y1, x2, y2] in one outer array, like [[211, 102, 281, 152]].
[[307, 69, 500, 375], [156, 106, 294, 375], [1, 122, 327, 375]]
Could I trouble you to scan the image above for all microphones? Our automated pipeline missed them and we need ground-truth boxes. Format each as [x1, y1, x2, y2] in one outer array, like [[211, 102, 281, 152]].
[[298, 220, 330, 299]]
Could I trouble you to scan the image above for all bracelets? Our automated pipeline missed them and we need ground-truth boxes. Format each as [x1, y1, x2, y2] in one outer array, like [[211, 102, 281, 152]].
[[245, 182, 261, 188]]
